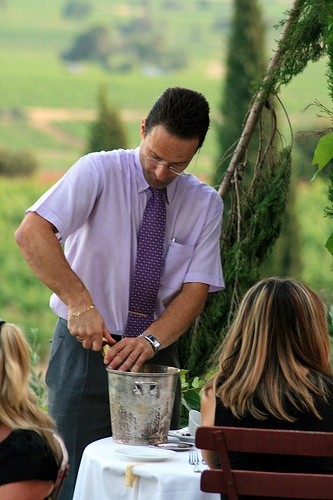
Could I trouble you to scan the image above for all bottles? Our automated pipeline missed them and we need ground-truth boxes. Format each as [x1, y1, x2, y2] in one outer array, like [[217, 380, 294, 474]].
[[49, 292, 131, 372]]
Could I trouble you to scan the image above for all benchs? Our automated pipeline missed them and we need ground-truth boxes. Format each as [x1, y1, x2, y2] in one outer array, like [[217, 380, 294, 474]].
[[193, 426, 333, 500]]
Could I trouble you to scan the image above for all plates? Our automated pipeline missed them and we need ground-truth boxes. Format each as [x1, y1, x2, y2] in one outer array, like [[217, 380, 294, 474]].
[[173, 430, 195, 442], [114, 446, 177, 461], [155, 441, 194, 451]]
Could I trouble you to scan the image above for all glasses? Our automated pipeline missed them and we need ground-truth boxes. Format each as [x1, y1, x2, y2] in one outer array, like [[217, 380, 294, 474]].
[[143, 126, 201, 178]]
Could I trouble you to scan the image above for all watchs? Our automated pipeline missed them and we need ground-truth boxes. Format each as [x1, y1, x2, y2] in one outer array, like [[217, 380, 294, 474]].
[[139, 333, 161, 357]]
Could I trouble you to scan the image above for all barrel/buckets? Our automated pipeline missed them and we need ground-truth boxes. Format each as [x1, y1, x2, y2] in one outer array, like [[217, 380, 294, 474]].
[[106, 362, 180, 445]]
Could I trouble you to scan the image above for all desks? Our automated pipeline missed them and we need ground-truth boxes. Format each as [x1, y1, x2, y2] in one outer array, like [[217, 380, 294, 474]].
[[70, 429, 220, 500]]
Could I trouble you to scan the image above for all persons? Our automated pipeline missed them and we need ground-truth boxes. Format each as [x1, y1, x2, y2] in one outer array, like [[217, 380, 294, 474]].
[[0, 321, 64, 500], [15, 88, 224, 500], [200, 277, 333, 500]]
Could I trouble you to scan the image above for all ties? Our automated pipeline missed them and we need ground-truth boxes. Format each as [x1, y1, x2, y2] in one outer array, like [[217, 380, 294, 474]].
[[123, 186, 170, 337]]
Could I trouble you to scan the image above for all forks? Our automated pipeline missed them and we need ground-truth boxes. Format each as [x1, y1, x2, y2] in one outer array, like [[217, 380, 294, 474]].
[[189, 452, 201, 472]]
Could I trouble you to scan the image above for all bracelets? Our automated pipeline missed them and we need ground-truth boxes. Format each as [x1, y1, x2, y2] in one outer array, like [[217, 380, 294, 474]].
[[68, 304, 96, 317]]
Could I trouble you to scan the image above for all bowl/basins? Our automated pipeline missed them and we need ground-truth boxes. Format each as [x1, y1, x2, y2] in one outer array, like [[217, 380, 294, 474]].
[[188, 410, 202, 435]]
